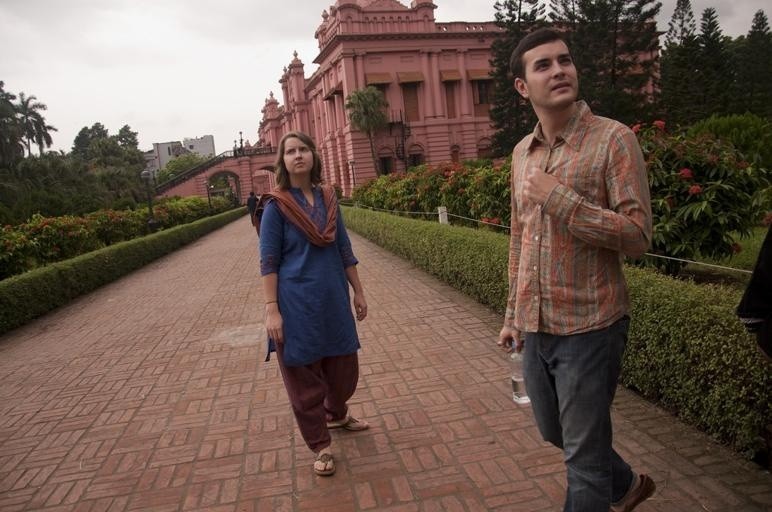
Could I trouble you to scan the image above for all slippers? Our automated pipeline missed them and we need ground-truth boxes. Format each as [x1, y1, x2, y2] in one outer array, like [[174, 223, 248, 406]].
[[325, 415, 370, 433], [312, 453, 338, 477]]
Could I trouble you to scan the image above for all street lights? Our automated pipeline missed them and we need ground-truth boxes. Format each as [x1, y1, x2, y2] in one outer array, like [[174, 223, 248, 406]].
[[239, 131, 243, 148], [348, 160, 356, 186], [203, 181, 214, 208], [140, 169, 157, 230], [234, 140, 237, 148]]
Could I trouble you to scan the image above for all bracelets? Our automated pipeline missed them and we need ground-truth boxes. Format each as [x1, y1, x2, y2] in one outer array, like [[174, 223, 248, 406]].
[[264, 299, 276, 307]]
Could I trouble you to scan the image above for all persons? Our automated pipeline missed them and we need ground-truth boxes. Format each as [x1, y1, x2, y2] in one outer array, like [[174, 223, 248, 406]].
[[246, 192, 256, 225], [259, 130, 370, 476], [495, 27, 660, 509]]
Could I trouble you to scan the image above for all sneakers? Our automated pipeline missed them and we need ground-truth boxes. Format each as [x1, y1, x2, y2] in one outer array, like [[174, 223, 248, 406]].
[[611, 471, 659, 512]]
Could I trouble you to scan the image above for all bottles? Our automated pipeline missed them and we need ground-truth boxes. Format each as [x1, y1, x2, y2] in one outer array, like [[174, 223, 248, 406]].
[[509, 340, 532, 406]]
